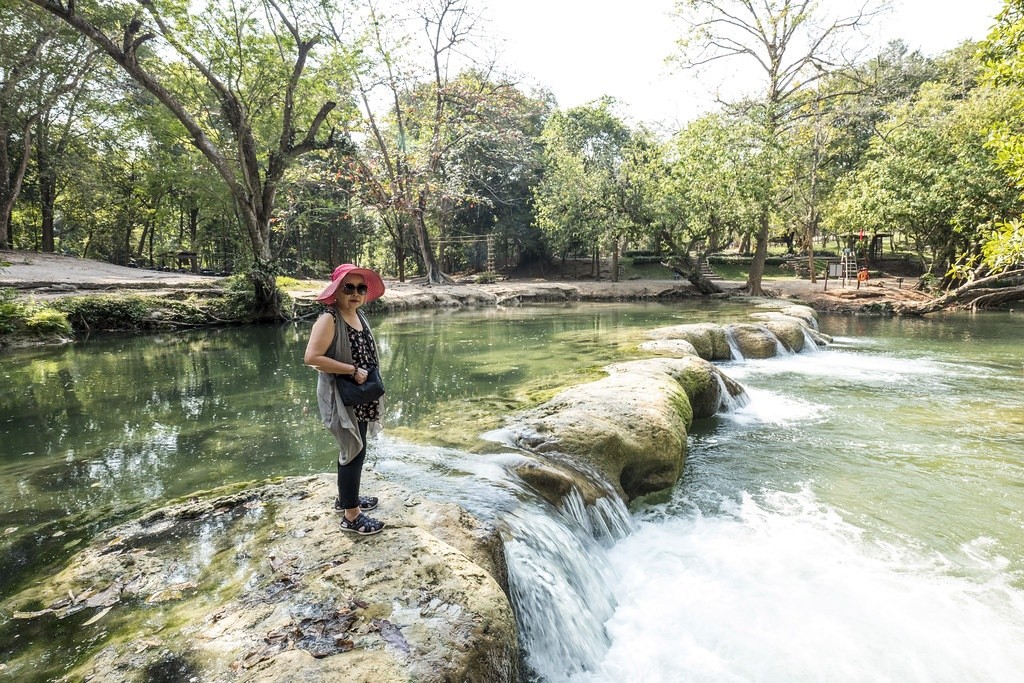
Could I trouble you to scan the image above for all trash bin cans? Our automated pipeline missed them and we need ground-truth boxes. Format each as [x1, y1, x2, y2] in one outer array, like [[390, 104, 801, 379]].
[[827, 260, 843, 279]]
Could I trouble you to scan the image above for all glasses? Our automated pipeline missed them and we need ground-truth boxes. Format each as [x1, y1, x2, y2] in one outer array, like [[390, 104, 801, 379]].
[[343, 283, 368, 295]]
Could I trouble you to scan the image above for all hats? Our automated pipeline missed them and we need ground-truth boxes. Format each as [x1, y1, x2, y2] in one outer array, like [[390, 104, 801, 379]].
[[317, 263, 385, 305]]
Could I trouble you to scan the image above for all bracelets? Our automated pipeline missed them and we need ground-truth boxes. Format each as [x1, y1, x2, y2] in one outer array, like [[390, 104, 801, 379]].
[[353, 363, 359, 376]]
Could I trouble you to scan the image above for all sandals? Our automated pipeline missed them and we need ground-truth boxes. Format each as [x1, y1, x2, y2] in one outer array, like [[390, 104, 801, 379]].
[[339, 513, 387, 535], [334, 496, 379, 512]]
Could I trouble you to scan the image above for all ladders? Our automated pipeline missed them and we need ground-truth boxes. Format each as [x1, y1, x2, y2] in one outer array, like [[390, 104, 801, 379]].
[[838, 249, 859, 288]]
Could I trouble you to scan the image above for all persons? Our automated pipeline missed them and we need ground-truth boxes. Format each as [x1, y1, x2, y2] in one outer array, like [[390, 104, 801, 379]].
[[303, 260, 386, 535]]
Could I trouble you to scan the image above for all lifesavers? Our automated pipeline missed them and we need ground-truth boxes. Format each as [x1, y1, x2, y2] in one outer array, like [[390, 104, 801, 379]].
[[857, 272, 866, 280]]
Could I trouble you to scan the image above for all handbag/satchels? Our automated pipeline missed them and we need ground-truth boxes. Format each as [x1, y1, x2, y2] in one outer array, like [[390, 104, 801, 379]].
[[334, 365, 385, 406]]
